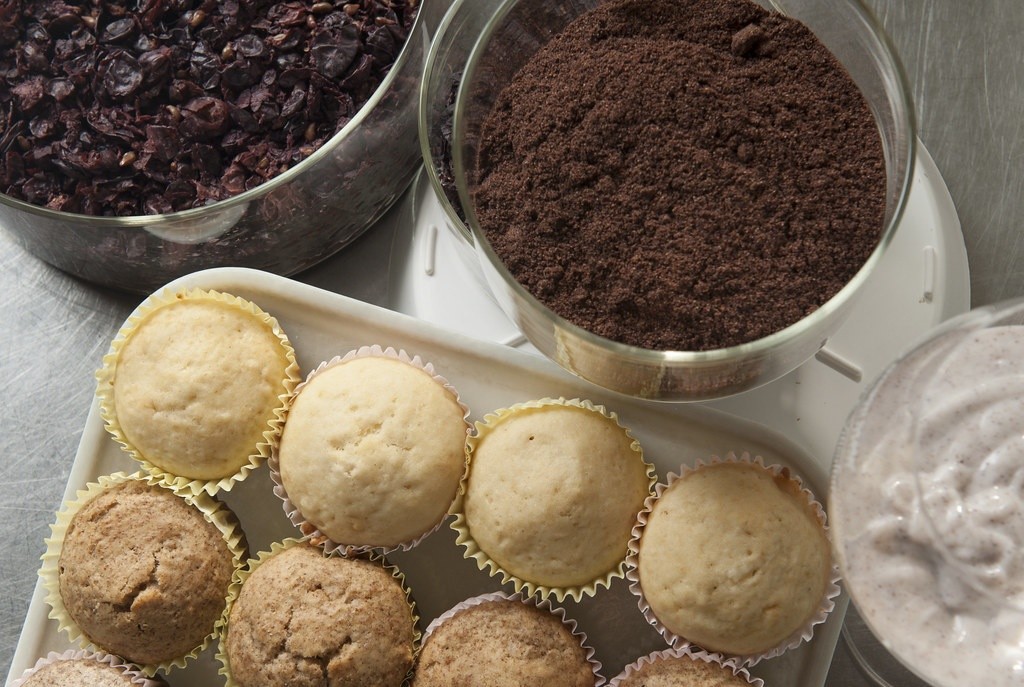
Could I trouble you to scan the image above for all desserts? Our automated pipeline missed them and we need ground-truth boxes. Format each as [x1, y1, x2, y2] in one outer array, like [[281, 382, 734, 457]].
[[19, 299, 828, 687]]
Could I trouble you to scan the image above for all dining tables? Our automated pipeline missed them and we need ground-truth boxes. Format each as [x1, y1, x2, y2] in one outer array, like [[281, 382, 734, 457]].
[[0, 117, 974, 685]]
